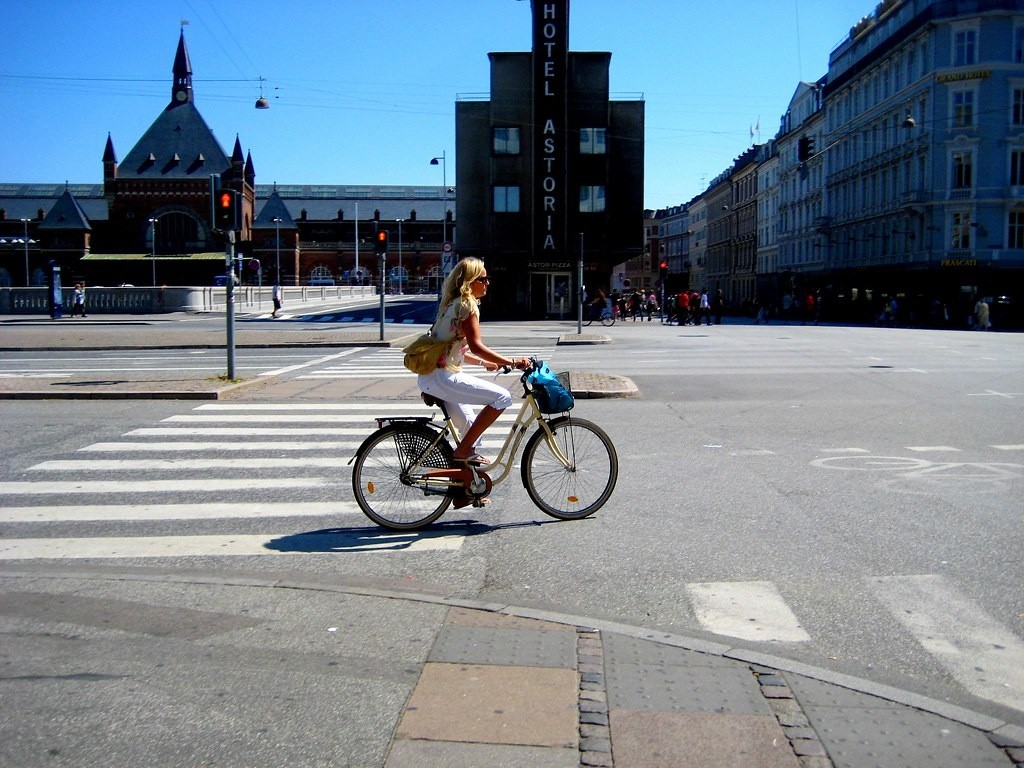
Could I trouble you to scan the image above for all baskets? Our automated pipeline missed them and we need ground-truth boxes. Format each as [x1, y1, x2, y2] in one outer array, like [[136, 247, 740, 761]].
[[531, 371, 574, 414]]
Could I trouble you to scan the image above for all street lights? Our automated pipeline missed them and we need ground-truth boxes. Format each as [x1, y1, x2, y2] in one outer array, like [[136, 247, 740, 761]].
[[429, 152, 447, 242], [272, 217, 284, 284], [19, 217, 31, 283], [148, 217, 159, 286]]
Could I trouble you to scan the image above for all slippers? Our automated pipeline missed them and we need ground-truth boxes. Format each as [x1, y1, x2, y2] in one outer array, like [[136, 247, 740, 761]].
[[454, 498, 490, 511], [452, 453, 493, 466]]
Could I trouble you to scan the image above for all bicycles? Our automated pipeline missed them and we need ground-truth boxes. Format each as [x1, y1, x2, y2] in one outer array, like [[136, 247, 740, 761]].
[[582, 303, 617, 328], [346, 352, 620, 532]]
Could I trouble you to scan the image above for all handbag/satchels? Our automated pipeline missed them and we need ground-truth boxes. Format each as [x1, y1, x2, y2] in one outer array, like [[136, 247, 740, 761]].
[[527, 360, 573, 412], [401, 334, 450, 374]]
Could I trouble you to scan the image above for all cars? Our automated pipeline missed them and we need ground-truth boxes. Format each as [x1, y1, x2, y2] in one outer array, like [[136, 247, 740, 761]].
[[305, 276, 336, 289]]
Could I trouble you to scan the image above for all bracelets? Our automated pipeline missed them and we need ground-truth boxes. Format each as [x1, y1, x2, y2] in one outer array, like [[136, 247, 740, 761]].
[[512, 358, 516, 367], [479, 360, 483, 365]]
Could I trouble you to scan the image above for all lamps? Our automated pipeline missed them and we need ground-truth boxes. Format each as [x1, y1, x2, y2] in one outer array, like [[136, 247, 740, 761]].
[[255, 81, 269, 109], [721, 203, 729, 211], [903, 114, 917, 128]]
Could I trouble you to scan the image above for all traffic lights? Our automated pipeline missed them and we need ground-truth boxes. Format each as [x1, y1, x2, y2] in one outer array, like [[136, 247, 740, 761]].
[[215, 190, 237, 230], [658, 261, 667, 281], [798, 137, 815, 162], [375, 230, 389, 254]]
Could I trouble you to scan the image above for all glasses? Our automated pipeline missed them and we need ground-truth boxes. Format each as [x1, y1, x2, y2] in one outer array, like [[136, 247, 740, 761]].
[[476, 276, 491, 283]]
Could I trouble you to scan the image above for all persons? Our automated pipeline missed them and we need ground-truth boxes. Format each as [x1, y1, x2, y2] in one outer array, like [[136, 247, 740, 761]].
[[271, 281, 282, 318], [755, 290, 990, 331], [587, 286, 723, 325], [416, 258, 534, 509], [70, 281, 88, 317]]
